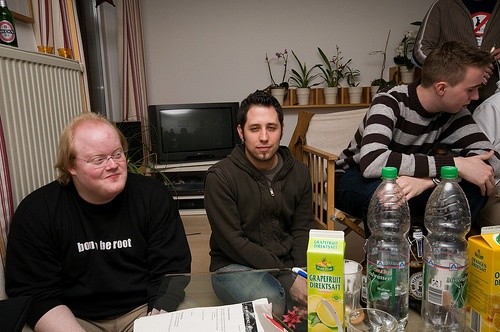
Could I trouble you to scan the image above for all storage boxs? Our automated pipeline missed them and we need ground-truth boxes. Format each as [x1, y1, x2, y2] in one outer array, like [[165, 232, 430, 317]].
[[465, 225, 500, 332], [306, 230, 346, 332]]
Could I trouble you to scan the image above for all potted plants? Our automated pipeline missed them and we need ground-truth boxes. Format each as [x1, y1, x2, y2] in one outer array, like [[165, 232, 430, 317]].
[[264, 49, 289, 105], [393, 32, 416, 84], [289, 49, 323, 105], [318, 44, 352, 105], [371, 79, 386, 101], [344, 66, 362, 104]]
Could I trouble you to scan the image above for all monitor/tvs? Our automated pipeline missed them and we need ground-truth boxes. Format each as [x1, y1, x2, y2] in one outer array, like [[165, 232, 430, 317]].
[[148, 102, 242, 164]]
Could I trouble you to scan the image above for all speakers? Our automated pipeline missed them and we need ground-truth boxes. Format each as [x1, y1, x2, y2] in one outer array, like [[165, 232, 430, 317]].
[[116, 121, 144, 169]]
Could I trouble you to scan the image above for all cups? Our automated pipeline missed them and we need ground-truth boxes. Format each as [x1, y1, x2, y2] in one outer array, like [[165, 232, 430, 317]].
[[57, 48, 72, 60], [347, 308, 398, 332], [37, 46, 54, 54], [344, 260, 363, 327]]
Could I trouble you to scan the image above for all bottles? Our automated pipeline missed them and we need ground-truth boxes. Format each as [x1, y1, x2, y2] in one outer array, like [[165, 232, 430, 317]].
[[0, 0, 18, 48], [359, 224, 400, 308], [366, 167, 410, 329], [408, 226, 432, 315], [421, 166, 471, 332]]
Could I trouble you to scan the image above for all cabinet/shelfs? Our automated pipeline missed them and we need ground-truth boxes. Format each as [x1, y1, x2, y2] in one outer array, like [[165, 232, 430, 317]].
[[145, 162, 216, 215]]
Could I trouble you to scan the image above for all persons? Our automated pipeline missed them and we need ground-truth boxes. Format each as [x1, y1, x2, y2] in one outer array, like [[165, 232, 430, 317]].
[[472, 80, 500, 226], [6, 113, 192, 332], [204, 90, 313, 316], [324, 39, 500, 237], [412, 0, 500, 114]]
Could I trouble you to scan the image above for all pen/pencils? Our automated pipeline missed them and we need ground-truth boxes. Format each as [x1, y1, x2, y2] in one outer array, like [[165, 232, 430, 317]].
[[292, 267, 307, 279], [490, 44, 496, 52], [265, 314, 288, 332]]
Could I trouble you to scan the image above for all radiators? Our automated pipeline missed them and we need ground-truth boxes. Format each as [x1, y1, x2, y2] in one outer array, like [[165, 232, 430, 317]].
[[0, 45, 85, 212]]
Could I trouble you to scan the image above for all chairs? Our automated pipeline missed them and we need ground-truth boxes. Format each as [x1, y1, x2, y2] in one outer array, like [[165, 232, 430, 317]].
[[298, 108, 365, 238]]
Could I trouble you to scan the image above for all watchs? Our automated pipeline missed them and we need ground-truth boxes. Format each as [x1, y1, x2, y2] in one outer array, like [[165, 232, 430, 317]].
[[432, 176, 441, 186]]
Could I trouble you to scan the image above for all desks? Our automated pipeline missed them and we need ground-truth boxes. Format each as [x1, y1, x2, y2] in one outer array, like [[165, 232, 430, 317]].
[[151, 267, 481, 332]]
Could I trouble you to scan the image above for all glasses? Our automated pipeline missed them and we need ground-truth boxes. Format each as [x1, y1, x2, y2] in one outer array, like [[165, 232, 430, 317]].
[[72, 150, 126, 168]]
